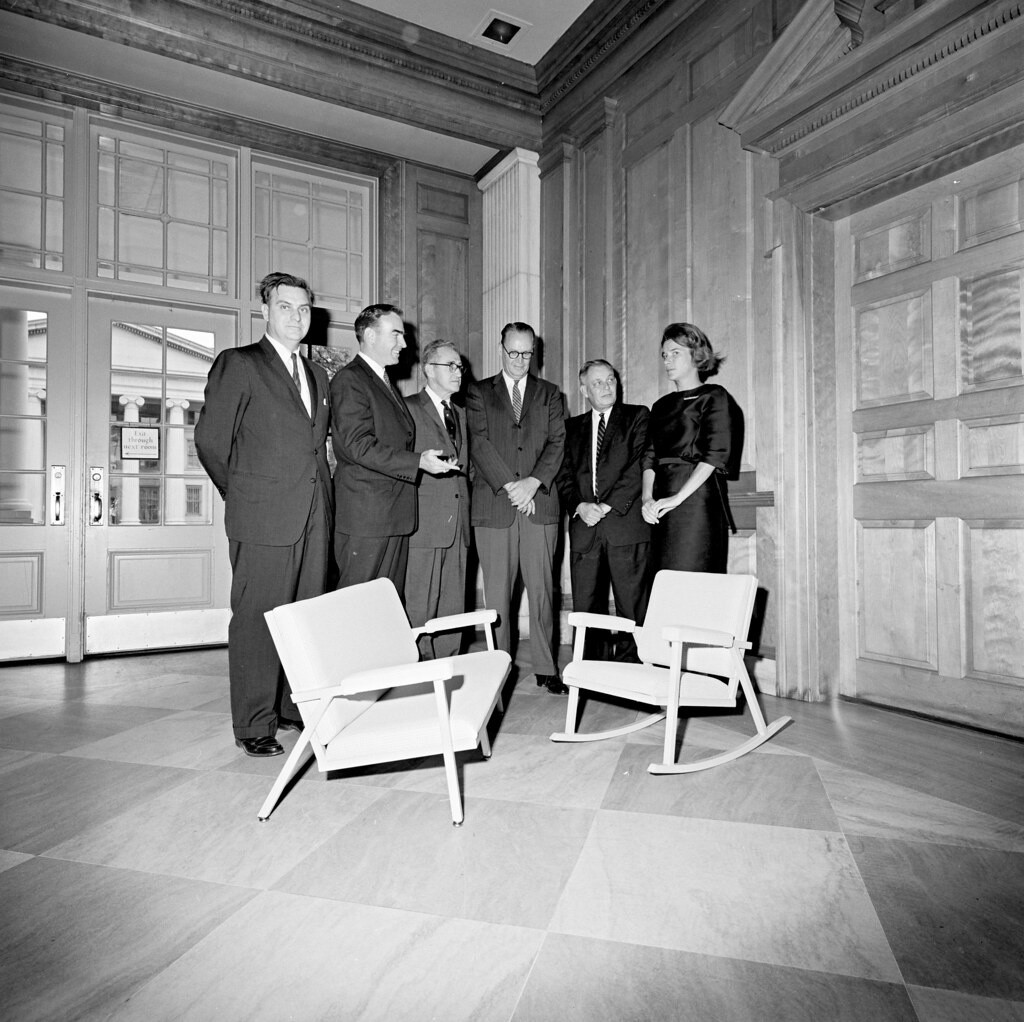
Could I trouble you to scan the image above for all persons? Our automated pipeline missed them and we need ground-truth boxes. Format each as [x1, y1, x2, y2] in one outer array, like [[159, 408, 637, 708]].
[[466, 321, 570, 695], [642, 322, 739, 627], [329, 304, 460, 605], [195, 272, 330, 757], [402, 339, 470, 662], [555, 359, 651, 664]]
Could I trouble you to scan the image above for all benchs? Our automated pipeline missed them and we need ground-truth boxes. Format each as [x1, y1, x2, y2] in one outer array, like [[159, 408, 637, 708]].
[[258, 577, 512, 826]]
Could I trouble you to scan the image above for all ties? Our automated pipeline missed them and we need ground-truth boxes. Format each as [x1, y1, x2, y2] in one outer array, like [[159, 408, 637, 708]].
[[291, 353, 300, 395], [596, 412, 607, 503], [512, 379, 522, 425], [441, 399, 456, 450], [383, 371, 405, 417]]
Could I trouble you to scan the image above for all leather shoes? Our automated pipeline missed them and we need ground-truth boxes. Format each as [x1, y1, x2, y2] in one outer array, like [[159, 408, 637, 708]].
[[234, 735, 284, 758], [277, 713, 305, 733], [536, 673, 570, 695]]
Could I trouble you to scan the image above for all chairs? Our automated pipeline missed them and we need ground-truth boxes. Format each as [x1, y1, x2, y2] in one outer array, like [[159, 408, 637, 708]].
[[548, 570, 792, 776]]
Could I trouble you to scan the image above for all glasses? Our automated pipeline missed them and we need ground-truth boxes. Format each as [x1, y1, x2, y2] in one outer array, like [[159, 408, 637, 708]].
[[500, 340, 533, 359], [429, 361, 467, 375]]
